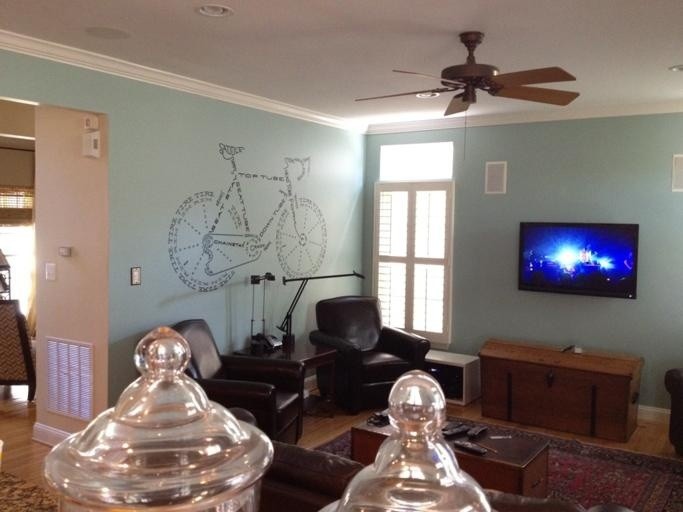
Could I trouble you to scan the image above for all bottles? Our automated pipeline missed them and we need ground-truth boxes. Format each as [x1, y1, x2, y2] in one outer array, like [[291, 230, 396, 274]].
[[320, 369, 494, 512], [42, 326, 275, 510]]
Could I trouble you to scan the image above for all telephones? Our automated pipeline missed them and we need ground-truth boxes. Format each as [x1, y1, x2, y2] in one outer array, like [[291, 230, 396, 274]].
[[258, 334, 283, 349]]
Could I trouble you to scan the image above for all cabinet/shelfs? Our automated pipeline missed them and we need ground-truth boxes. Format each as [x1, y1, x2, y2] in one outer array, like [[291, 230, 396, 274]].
[[423, 350, 481, 406]]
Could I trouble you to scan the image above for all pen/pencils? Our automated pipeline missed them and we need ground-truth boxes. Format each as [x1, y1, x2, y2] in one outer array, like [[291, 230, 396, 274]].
[[473, 441, 497, 453]]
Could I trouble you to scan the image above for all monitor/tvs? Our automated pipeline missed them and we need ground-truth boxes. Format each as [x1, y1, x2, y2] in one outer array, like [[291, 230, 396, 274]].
[[519, 222, 638, 300]]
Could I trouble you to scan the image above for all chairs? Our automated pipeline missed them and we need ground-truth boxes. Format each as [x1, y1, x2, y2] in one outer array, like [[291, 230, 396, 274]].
[[310, 294, 431, 414], [172, 318, 306, 444]]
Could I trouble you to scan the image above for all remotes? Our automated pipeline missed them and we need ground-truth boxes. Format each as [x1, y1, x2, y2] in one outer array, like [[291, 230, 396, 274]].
[[442, 420, 489, 454]]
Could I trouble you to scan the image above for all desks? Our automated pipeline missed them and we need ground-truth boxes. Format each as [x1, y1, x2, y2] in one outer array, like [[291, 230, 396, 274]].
[[478, 339, 643, 442]]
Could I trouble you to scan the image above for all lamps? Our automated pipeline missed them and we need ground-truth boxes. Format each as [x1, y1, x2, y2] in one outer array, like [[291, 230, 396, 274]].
[[250, 273, 275, 355], [273, 268, 365, 348]]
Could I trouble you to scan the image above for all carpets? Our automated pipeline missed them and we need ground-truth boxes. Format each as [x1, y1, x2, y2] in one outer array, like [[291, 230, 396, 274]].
[[309, 414, 683, 512]]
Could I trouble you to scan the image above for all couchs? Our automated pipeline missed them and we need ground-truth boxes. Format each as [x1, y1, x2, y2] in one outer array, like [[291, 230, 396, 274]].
[[262, 440, 635, 512]]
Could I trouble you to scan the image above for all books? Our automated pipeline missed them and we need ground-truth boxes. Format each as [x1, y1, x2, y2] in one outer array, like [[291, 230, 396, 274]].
[[0, 248, 10, 292]]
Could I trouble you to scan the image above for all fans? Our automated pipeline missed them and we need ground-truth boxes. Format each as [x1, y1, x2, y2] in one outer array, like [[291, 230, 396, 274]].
[[353, 31, 580, 116]]
